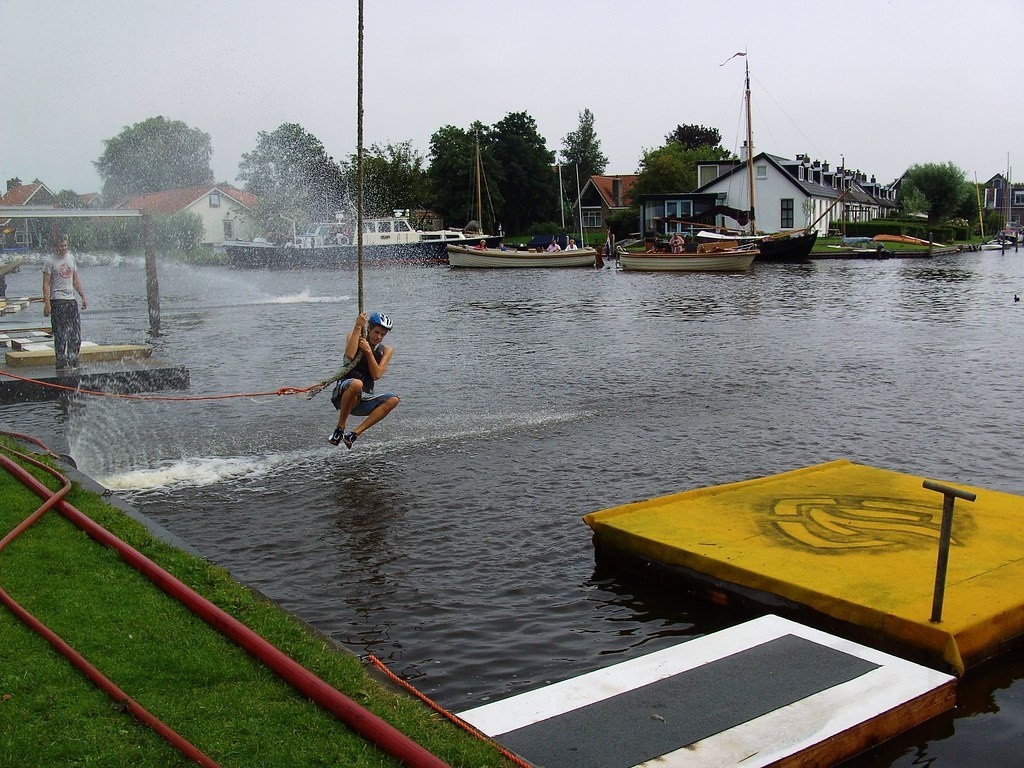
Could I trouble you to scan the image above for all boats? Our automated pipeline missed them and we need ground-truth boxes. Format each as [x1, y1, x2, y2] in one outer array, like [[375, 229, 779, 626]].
[[414, 229, 505, 263], [222, 189, 426, 269], [446, 240, 598, 270], [653, 45, 818, 265], [618, 250, 761, 272]]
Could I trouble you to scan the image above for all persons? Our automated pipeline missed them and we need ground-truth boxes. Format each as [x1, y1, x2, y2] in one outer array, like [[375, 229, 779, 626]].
[[40, 232, 88, 373], [669, 232, 685, 253], [546, 239, 561, 252], [564, 238, 579, 252], [327, 311, 401, 450], [474, 238, 488, 251]]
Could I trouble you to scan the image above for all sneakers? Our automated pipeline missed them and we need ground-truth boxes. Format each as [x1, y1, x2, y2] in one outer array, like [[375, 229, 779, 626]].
[[328, 426, 344, 446], [343, 431, 357, 450]]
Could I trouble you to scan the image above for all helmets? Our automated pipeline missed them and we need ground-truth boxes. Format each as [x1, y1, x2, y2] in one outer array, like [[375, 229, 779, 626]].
[[368, 313, 393, 331]]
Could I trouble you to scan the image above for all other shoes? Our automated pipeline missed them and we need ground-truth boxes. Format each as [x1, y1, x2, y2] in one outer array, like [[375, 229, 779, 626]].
[[68, 361, 87, 369], [55, 364, 77, 371]]
